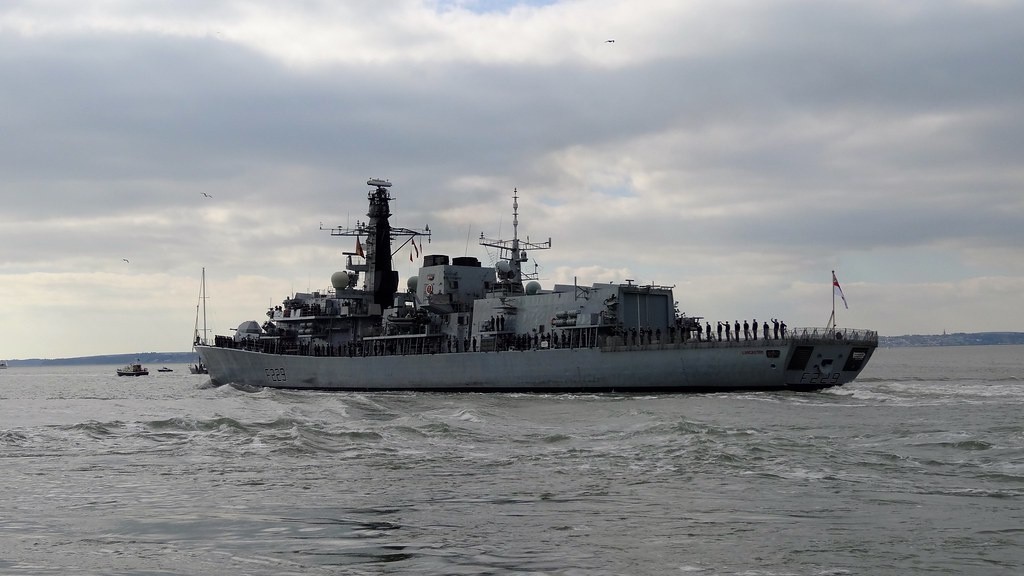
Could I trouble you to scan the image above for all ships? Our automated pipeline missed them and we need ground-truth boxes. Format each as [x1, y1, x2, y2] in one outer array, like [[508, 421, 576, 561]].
[[194, 178, 879, 395]]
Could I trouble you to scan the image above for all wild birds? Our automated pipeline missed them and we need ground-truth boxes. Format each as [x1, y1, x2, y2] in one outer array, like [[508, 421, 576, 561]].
[[123, 258, 129, 263], [199, 192, 214, 199], [604, 40, 616, 43]]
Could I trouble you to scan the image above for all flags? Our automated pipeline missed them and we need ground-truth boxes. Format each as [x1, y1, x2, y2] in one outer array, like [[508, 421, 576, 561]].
[[357, 238, 365, 259], [833, 274, 848, 309]]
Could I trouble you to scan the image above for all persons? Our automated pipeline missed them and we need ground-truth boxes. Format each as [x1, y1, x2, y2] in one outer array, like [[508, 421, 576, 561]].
[[276, 341, 297, 355], [500, 315, 505, 331], [300, 340, 309, 355], [338, 342, 341, 356], [390, 342, 430, 355], [491, 316, 495, 331], [496, 316, 499, 331], [266, 290, 448, 336], [498, 319, 787, 351], [473, 337, 477, 352], [463, 337, 468, 352], [455, 338, 459, 353], [331, 344, 333, 355], [314, 343, 321, 357], [322, 344, 325, 356], [343, 341, 384, 357], [271, 341, 275, 354], [326, 344, 330, 356], [448, 339, 451, 353], [215, 335, 264, 349]]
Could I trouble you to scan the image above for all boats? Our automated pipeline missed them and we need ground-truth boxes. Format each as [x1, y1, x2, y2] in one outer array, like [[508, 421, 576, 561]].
[[387, 309, 431, 327], [158, 367, 173, 372], [188, 364, 208, 374], [0, 363, 8, 369], [116, 364, 149, 376]]
